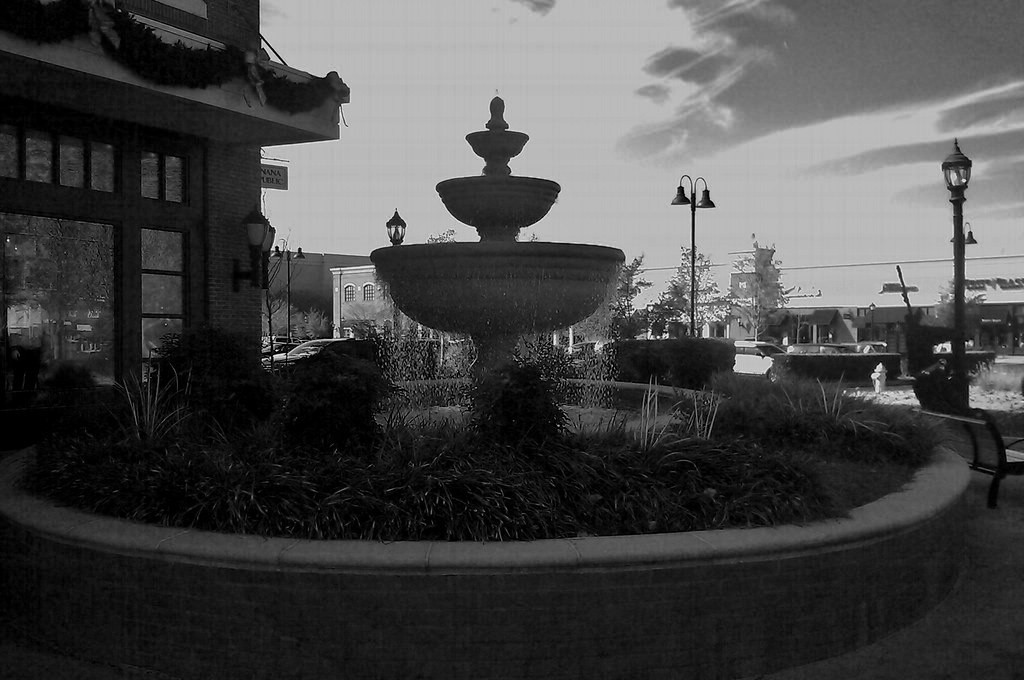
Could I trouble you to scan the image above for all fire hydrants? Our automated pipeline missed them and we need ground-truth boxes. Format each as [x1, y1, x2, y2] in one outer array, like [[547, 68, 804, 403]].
[[870, 363, 887, 393]]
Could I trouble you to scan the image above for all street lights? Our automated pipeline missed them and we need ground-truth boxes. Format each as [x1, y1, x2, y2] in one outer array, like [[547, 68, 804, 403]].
[[670, 175, 716, 337], [270, 238, 305, 345], [940, 138, 977, 407]]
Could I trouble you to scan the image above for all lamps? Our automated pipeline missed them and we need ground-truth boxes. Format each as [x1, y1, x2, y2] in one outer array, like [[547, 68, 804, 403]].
[[262, 224, 275, 289], [230, 210, 269, 292]]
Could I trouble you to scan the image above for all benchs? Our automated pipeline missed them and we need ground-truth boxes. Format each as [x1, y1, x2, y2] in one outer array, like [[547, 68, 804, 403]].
[[910, 407, 1024, 510]]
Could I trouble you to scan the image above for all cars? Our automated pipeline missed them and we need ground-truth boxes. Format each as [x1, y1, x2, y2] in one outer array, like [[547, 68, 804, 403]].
[[733, 340, 787, 383], [261, 336, 349, 373]]
